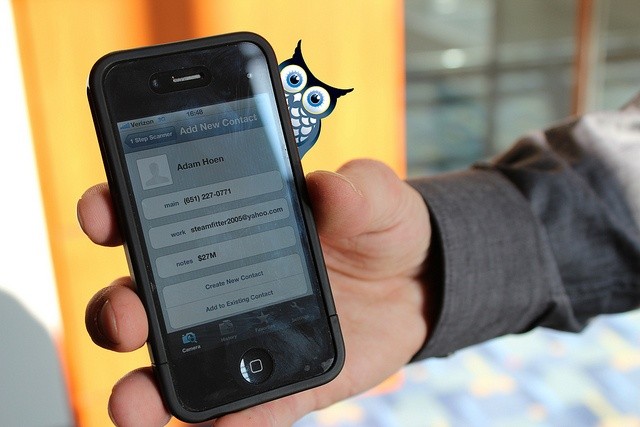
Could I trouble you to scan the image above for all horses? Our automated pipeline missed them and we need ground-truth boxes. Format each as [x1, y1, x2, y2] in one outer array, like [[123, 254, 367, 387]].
[[86, 30, 345, 423]]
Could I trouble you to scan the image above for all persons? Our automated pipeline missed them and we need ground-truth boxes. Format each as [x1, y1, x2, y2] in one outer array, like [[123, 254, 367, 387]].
[[77, 94, 640, 427]]
